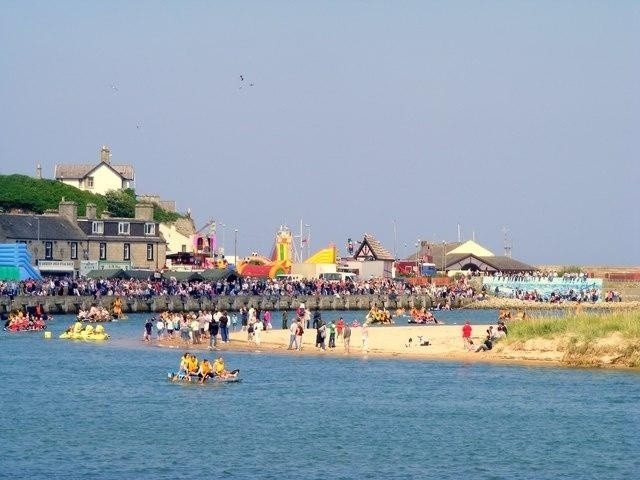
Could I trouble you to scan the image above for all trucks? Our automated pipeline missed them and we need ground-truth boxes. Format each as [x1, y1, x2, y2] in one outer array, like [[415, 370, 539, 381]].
[[319, 272, 369, 290]]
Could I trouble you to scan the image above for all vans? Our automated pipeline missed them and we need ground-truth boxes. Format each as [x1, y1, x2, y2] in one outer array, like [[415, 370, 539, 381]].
[[277, 274, 301, 283]]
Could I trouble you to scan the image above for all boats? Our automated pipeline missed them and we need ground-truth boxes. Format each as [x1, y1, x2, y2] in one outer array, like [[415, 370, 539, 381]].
[[60, 330, 111, 342], [166, 369, 239, 381], [364, 319, 396, 327], [407, 318, 437, 324], [4, 320, 48, 332], [76, 312, 115, 324]]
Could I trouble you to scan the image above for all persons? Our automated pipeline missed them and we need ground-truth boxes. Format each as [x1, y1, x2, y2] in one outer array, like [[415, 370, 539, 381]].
[[1, 273, 151, 297], [462, 321, 473, 351], [404, 280, 485, 310], [365, 304, 394, 326], [409, 305, 439, 325], [495, 287, 616, 303], [142, 309, 230, 351], [232, 299, 270, 345], [265, 272, 408, 295], [475, 321, 508, 352], [3, 301, 48, 331], [180, 352, 238, 378], [71, 319, 105, 333], [77, 297, 124, 320], [472, 270, 589, 284], [282, 309, 288, 330], [286, 302, 352, 353], [148, 275, 268, 300]]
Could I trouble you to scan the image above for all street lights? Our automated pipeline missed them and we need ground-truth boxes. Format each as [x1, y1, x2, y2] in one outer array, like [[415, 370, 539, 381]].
[[392, 216, 399, 257], [217, 222, 226, 259], [415, 244, 418, 266], [33, 216, 41, 266], [232, 227, 239, 266], [405, 244, 407, 259], [417, 239, 420, 267], [442, 240, 445, 271], [444, 241, 447, 273]]
